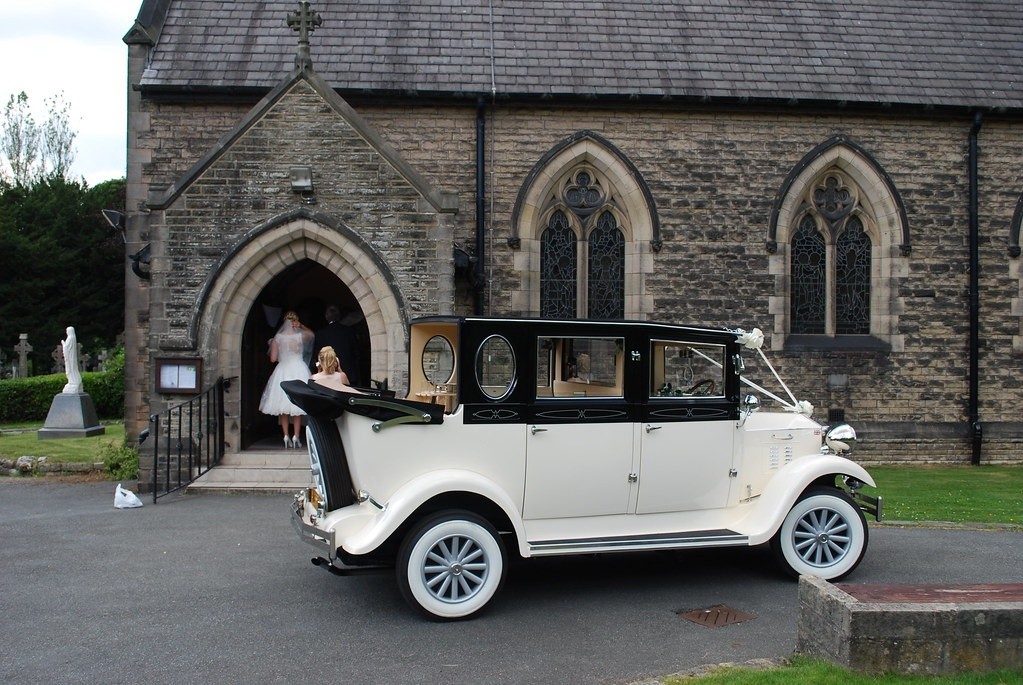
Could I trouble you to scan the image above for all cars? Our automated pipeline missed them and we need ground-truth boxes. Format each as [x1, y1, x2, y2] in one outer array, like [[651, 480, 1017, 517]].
[[281, 316, 883, 618]]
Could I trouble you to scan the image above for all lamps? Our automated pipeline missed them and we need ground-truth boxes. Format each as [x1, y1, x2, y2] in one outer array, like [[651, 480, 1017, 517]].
[[101, 209, 125, 231], [289, 166, 316, 204]]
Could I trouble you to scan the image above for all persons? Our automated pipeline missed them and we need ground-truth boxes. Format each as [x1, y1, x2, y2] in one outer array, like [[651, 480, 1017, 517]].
[[61, 325, 82, 386], [572, 353, 594, 380], [258, 311, 315, 449], [311, 345, 350, 385]]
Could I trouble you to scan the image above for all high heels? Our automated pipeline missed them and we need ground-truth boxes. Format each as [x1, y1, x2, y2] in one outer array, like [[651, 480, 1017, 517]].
[[292, 435, 302, 448], [284, 434, 292, 448]]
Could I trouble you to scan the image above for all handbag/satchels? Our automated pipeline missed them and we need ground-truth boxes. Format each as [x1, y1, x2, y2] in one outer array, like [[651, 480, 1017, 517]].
[[114, 483, 143, 508]]
